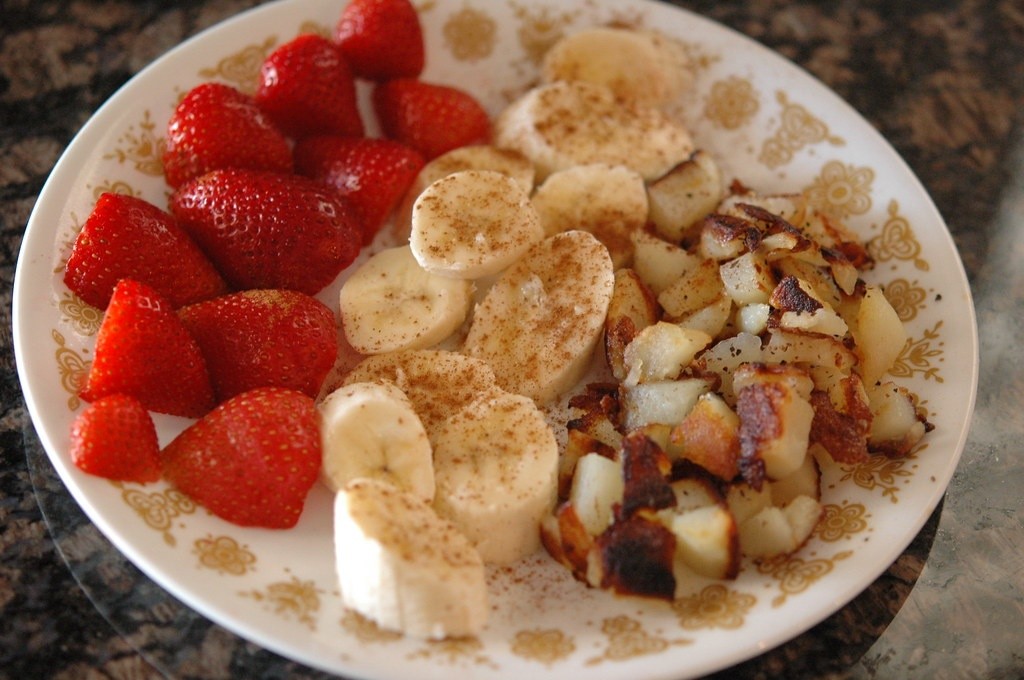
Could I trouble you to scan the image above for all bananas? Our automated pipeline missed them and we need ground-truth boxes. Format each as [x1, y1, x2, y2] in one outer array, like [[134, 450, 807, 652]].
[[318, 30, 700, 641]]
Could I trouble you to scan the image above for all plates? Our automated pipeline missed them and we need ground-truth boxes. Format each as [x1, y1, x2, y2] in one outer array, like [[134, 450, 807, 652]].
[[13, 0, 978, 679]]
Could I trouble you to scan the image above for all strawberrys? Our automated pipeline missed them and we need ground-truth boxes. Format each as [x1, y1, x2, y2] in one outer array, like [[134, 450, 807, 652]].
[[64, 1, 486, 531]]
